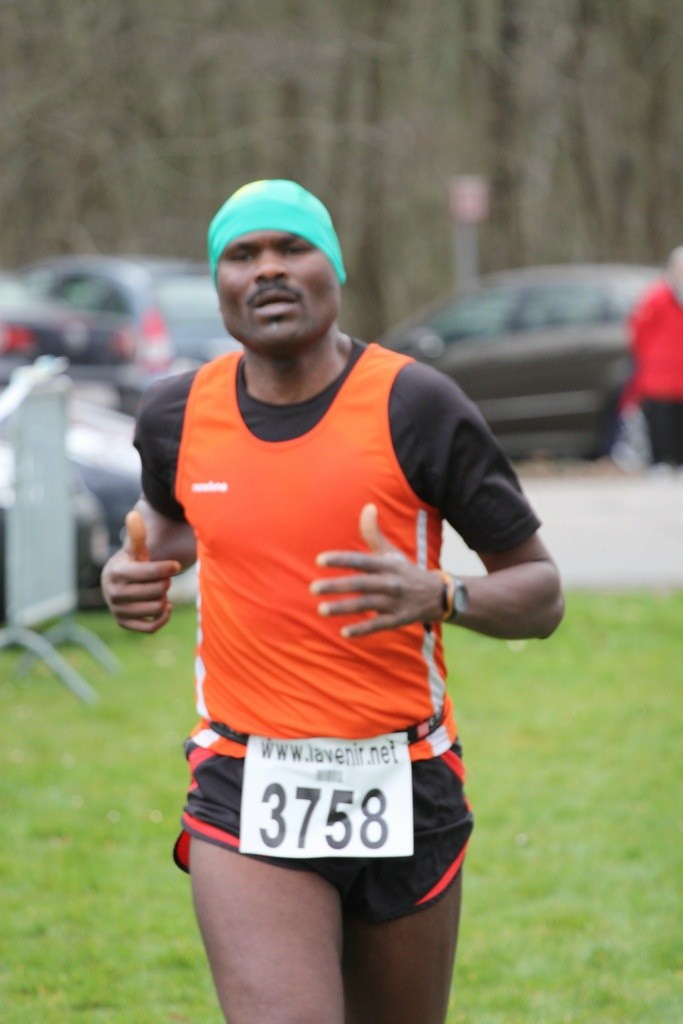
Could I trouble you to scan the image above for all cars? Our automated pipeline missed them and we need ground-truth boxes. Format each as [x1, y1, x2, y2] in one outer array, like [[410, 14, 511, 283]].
[[0, 256, 244, 416], [371, 263, 669, 466]]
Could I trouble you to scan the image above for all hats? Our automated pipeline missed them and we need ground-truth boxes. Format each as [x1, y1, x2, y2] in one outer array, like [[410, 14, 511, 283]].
[[205, 179, 347, 285]]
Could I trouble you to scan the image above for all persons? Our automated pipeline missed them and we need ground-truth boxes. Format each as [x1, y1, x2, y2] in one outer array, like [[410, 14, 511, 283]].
[[101, 179, 566, 1024], [591, 247, 682, 467]]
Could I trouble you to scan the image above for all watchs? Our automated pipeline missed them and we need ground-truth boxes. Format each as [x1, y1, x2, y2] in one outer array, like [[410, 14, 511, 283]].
[[423, 570, 468, 633]]
